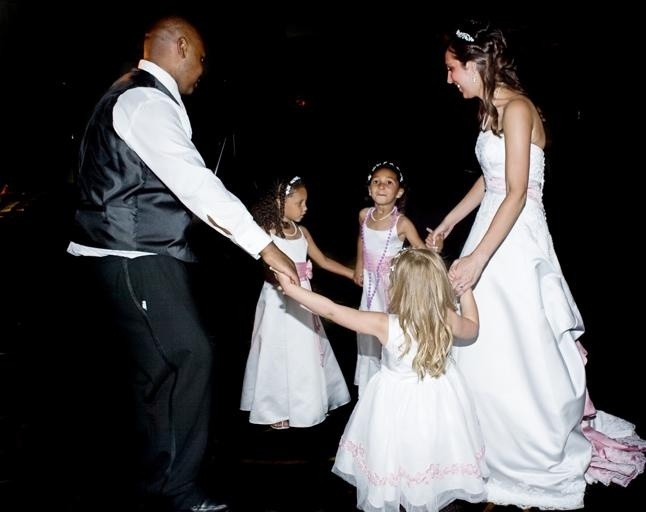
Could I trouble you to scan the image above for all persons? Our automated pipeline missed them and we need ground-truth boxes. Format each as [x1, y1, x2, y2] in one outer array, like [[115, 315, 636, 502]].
[[238, 173, 354, 431], [270, 248, 489, 512], [353, 160, 444, 400], [66, 17, 302, 512], [425, 19, 645, 512]]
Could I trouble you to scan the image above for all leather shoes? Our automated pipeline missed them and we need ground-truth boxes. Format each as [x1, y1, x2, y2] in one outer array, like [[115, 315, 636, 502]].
[[190, 497, 227, 511]]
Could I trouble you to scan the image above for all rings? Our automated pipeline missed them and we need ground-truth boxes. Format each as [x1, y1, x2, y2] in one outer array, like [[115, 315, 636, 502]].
[[456, 283, 464, 289]]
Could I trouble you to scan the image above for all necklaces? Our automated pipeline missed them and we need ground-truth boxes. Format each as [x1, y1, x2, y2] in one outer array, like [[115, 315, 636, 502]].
[[280, 220, 298, 237], [370, 208, 395, 222], [481, 82, 505, 130]]
